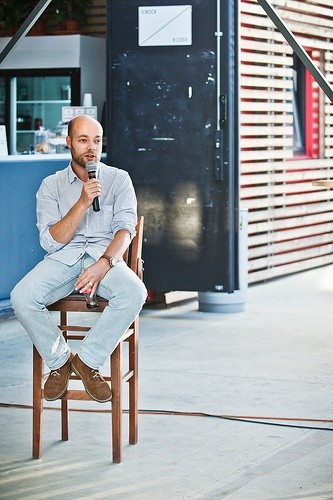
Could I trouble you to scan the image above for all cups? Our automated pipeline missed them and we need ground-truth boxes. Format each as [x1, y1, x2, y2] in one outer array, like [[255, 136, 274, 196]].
[[83, 92, 93, 106]]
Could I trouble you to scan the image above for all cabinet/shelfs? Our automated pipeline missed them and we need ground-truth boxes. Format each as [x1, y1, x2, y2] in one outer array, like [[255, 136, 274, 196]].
[[0, 35, 108, 153]]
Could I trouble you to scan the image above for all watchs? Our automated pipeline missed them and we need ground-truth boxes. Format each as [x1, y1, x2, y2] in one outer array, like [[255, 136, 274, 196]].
[[99, 255, 117, 267]]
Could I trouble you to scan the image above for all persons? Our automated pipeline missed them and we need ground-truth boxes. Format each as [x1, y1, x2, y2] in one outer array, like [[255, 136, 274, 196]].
[[9, 115, 148, 403]]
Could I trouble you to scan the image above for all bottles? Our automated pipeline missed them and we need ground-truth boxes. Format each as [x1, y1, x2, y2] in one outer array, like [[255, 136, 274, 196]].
[[80, 268, 99, 309]]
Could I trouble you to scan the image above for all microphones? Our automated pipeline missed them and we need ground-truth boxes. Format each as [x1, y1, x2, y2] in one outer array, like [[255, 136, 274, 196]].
[[86, 161, 100, 212]]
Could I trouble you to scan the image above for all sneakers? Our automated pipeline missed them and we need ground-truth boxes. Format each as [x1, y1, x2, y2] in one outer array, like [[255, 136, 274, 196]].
[[71, 353, 112, 403], [43, 353, 74, 401]]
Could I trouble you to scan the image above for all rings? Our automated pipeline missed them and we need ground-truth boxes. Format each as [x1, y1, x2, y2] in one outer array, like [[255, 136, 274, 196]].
[[88, 281, 93, 287]]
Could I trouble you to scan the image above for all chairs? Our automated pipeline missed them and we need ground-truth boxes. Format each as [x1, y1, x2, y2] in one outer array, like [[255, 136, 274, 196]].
[[32, 215, 144, 464]]
